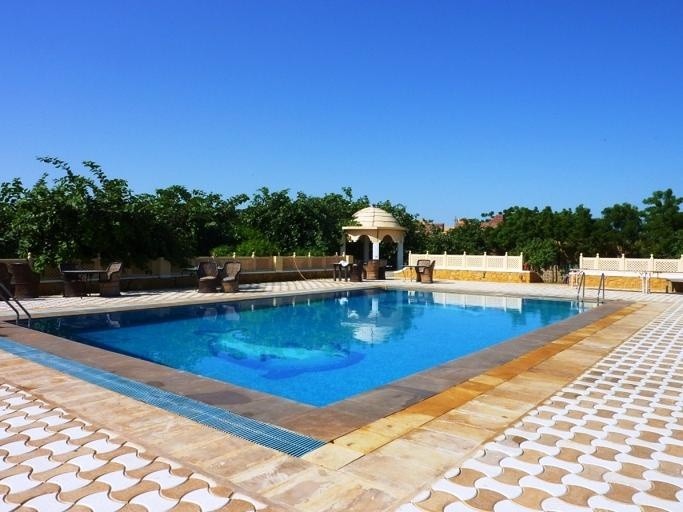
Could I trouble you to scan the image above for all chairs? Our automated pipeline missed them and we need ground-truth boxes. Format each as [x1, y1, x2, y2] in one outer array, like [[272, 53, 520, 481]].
[[9, 263, 40, 301], [56, 262, 86, 296], [97, 261, 123, 296], [415, 259, 435, 284], [348, 258, 387, 281], [196, 261, 241, 292]]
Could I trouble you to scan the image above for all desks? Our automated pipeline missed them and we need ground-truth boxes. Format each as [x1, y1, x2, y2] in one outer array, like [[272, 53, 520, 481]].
[[61, 269, 107, 299], [397, 265, 426, 282], [179, 266, 198, 290], [329, 262, 351, 282]]
